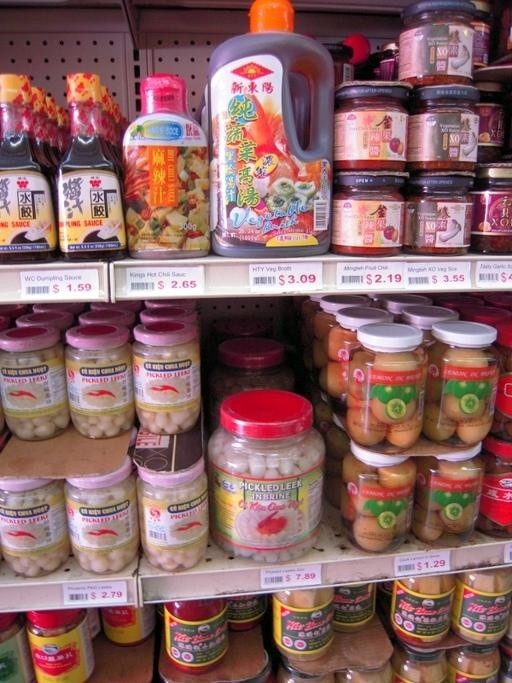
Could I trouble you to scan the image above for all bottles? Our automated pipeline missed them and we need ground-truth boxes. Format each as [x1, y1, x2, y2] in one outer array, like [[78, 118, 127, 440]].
[[0, 73, 210, 261]]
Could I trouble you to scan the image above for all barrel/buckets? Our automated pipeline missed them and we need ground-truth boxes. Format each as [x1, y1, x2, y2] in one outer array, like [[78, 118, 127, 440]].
[[208, 0, 335, 258]]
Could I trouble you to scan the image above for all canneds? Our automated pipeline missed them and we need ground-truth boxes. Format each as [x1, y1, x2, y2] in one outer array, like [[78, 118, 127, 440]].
[[275, 567, 512, 682], [207, 314, 325, 558], [301, 290, 512, 553], [331, 1, 512, 257], [0, 300, 210, 573], [1, 594, 268, 683]]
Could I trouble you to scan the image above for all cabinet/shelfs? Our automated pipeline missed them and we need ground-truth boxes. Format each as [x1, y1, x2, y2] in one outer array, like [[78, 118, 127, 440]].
[[0, 0, 512, 616]]
